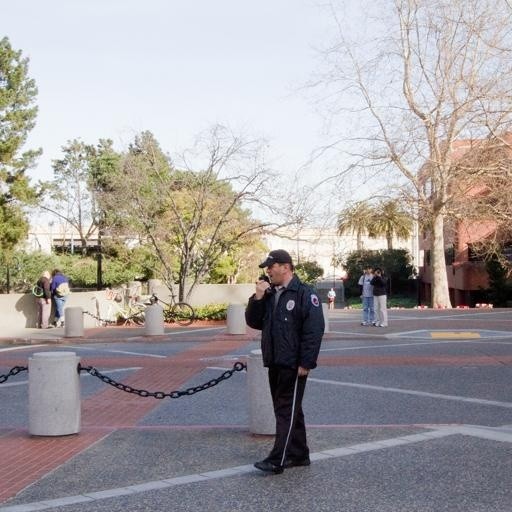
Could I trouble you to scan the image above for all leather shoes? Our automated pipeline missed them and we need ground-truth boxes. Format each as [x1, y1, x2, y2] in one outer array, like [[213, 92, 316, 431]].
[[284, 458, 311, 466], [255, 461, 284, 474]]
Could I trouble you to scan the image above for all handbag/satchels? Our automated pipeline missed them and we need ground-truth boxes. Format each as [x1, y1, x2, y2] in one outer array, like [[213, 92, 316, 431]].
[[33, 285, 44, 297], [56, 282, 70, 297]]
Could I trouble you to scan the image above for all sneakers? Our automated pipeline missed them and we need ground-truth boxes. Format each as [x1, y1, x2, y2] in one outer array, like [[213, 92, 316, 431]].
[[361, 321, 386, 327], [36, 321, 65, 328]]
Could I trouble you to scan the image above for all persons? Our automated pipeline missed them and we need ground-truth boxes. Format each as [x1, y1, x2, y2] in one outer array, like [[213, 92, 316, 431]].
[[33, 270, 55, 329], [243, 249, 326, 475], [50, 268, 72, 328], [358, 264, 374, 325], [369, 268, 389, 328], [326, 287, 337, 309]]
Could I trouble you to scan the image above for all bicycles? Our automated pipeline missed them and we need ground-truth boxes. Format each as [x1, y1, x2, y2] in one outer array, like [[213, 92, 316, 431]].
[[101, 287, 195, 328]]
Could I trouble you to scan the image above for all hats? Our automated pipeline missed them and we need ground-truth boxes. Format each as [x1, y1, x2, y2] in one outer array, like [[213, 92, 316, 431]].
[[259, 250, 291, 268]]
